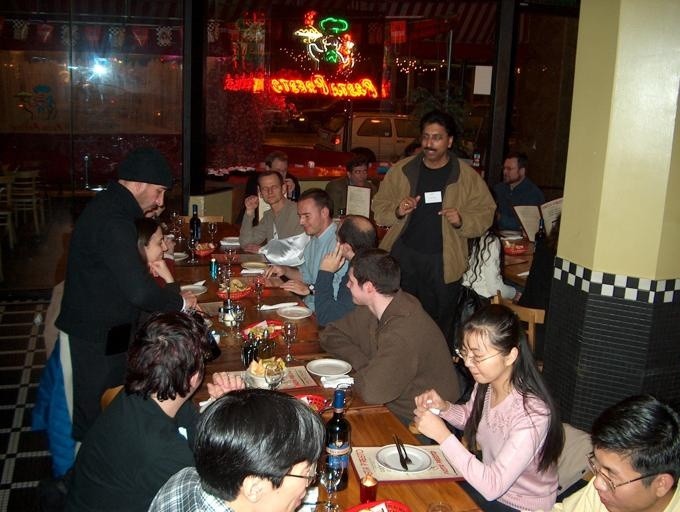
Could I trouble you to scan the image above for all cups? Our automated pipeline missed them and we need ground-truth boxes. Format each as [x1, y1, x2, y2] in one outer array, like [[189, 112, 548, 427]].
[[264, 364, 284, 391], [318, 455, 343, 510], [230, 301, 247, 339], [255, 275, 266, 299], [281, 321, 298, 362], [335, 383, 354, 412], [187, 237, 198, 263]]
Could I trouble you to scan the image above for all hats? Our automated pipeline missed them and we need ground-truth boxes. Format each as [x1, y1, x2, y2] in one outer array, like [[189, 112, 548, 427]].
[[117, 145, 177, 186]]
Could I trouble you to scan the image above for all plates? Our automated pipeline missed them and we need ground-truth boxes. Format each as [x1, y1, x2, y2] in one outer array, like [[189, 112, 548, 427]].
[[216, 290, 251, 300], [306, 358, 352, 376], [276, 306, 312, 320], [375, 445, 434, 474], [242, 319, 283, 342], [241, 261, 266, 269], [194, 243, 216, 256], [174, 252, 189, 261], [180, 285, 208, 296], [348, 501, 410, 511], [247, 364, 289, 380], [294, 394, 330, 414]]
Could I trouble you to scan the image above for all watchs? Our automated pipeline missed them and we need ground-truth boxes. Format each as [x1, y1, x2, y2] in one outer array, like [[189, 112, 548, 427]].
[[308, 283, 314, 295]]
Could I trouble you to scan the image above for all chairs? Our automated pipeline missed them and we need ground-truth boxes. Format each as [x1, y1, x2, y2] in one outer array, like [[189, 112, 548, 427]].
[[486, 299, 545, 373], [0, 177, 17, 249], [11, 171, 45, 235]]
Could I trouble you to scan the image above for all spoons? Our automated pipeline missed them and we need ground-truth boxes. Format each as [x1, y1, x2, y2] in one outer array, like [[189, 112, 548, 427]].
[[398, 439, 412, 464]]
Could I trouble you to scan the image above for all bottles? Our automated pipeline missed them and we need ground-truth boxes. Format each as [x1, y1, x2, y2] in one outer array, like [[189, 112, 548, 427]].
[[189, 204, 201, 241], [325, 390, 352, 491], [359, 472, 378, 504]]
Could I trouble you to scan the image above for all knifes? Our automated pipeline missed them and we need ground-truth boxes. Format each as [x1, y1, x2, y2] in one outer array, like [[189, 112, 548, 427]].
[[392, 434, 408, 470]]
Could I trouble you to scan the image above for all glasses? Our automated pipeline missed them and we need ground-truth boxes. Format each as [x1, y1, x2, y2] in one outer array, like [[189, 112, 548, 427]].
[[501, 165, 516, 171], [287, 465, 316, 487], [584, 451, 659, 494], [455, 348, 504, 365]]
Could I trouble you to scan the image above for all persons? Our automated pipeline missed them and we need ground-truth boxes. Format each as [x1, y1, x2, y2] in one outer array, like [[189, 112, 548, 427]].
[[239, 171, 304, 254], [550, 393, 680, 512], [461, 213, 522, 303], [314, 214, 376, 325], [62, 310, 248, 512], [318, 249, 461, 445], [137, 219, 181, 291], [52, 147, 197, 422], [413, 305, 566, 512], [494, 152, 543, 230], [371, 110, 497, 324], [263, 187, 350, 313], [148, 388, 326, 512], [234, 151, 300, 227], [324, 159, 376, 217]]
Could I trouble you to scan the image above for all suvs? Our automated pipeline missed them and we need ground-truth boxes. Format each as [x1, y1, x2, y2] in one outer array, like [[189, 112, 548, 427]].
[[312, 113, 474, 166]]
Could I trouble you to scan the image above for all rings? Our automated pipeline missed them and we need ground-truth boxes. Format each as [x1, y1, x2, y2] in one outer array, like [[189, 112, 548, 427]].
[[404, 202, 409, 207]]
